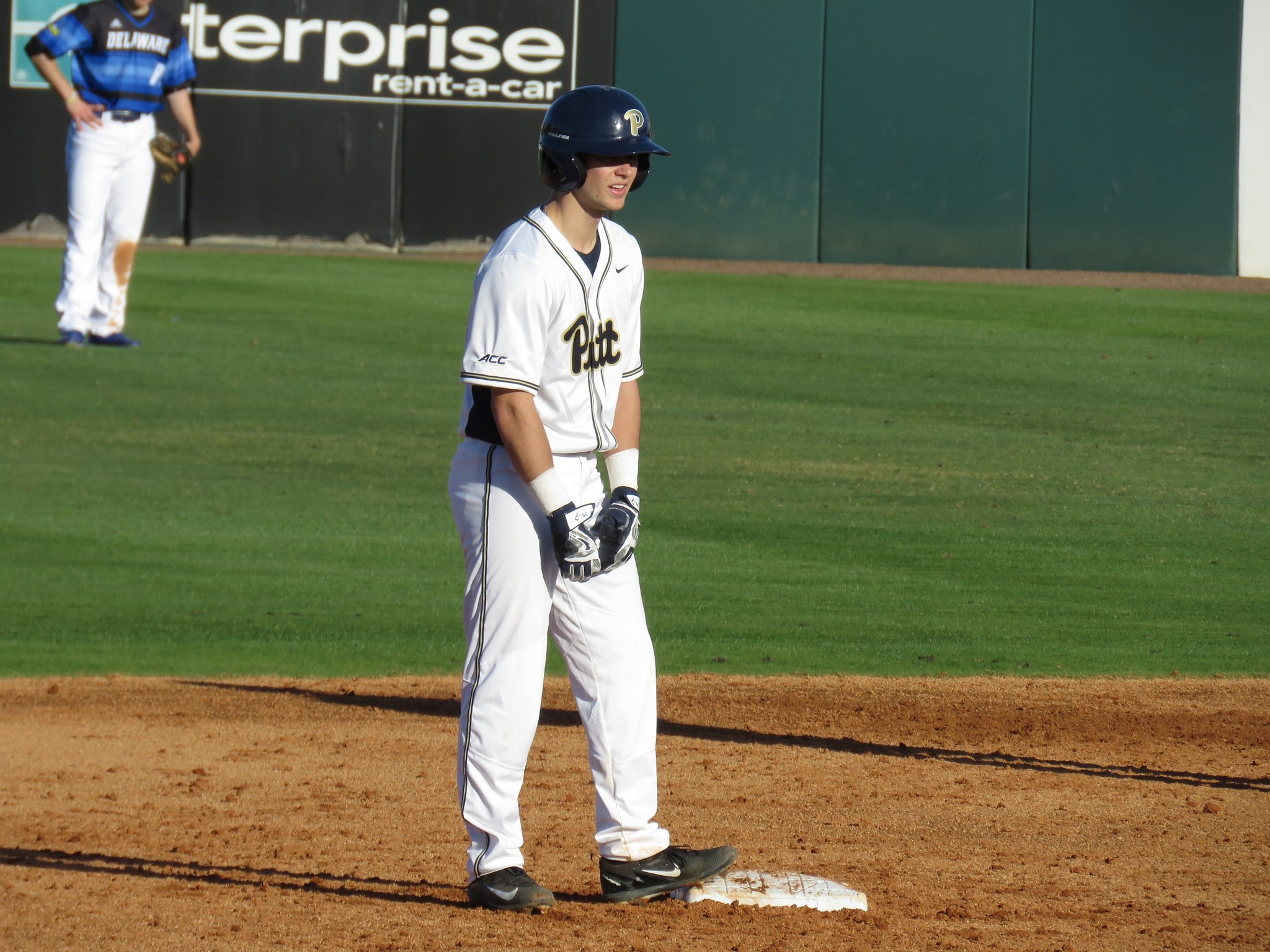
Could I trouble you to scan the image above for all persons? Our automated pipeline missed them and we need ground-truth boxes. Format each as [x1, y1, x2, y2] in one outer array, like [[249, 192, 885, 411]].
[[25, 0, 204, 349], [444, 85, 737, 911]]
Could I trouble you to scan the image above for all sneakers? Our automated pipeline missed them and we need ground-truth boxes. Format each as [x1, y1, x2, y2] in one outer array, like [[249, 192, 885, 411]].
[[465, 867, 554, 912], [598, 844, 738, 903]]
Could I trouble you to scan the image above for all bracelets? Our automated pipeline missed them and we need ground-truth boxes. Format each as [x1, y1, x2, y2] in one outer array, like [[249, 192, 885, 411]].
[[63, 90, 78, 105]]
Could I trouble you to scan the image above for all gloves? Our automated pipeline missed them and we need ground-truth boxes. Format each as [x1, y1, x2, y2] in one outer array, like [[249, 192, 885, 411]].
[[590, 489, 641, 572], [149, 132, 195, 185], [546, 501, 601, 583]]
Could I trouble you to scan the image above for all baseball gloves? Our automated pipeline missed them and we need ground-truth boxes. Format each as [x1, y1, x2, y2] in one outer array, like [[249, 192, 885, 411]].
[[149, 136, 193, 182]]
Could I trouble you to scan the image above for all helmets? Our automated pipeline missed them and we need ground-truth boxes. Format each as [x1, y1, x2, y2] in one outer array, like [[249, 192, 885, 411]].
[[537, 82, 672, 192]]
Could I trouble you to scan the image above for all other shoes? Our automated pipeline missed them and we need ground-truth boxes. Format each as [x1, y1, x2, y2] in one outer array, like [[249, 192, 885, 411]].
[[86, 330, 139, 349], [59, 327, 84, 349]]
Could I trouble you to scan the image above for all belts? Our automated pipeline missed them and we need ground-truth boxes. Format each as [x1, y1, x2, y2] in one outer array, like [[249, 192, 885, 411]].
[[109, 109, 140, 122]]
